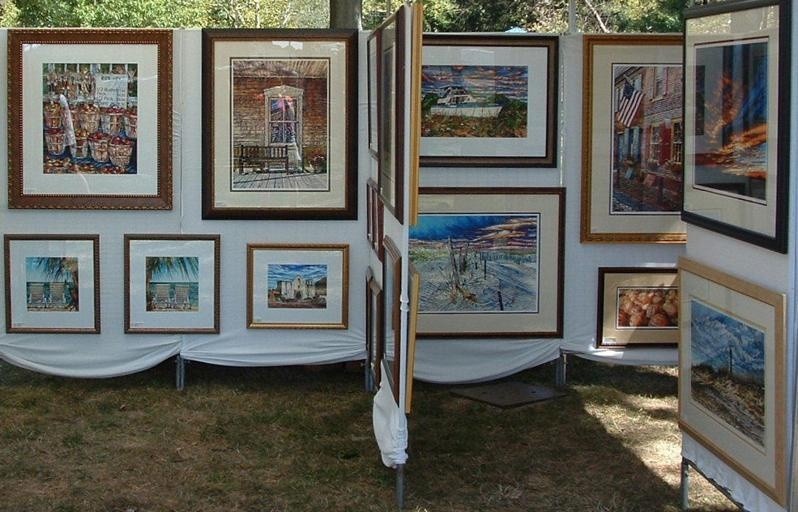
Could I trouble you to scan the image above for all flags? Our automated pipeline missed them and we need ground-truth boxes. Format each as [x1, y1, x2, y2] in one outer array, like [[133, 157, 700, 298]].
[[615, 73, 647, 130]]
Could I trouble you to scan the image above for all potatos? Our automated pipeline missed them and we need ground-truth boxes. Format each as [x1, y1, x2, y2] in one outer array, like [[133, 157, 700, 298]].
[[619, 290, 679, 327]]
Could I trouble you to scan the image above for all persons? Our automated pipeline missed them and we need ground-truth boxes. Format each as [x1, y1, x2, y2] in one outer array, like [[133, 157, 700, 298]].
[[699, 57, 744, 151]]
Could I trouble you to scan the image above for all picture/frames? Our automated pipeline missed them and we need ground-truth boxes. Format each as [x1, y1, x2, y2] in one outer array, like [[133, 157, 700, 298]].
[[246, 242, 349, 331], [379, 5, 406, 224], [381, 234, 402, 408], [3, 232, 101, 336], [362, 265, 383, 391], [418, 33, 560, 169], [369, 23, 378, 157], [6, 26, 174, 213], [595, 266, 679, 353], [580, 33, 685, 245], [366, 175, 383, 261], [412, 186, 568, 337], [678, 255, 789, 510], [677, 0, 791, 257], [122, 233, 222, 337]]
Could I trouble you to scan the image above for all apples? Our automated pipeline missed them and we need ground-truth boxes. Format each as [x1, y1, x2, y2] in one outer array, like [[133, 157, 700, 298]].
[[42, 101, 137, 175]]
